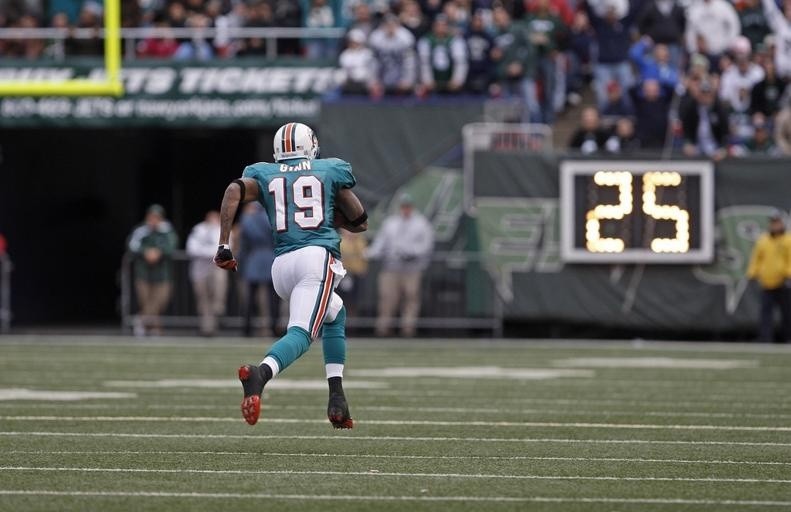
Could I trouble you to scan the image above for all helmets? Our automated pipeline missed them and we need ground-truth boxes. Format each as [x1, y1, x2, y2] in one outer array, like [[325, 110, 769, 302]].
[[273, 122, 318, 163]]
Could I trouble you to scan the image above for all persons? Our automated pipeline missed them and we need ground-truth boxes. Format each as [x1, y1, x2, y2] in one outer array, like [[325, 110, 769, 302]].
[[745, 210, 791, 347], [340, 0, 791, 162], [127, 202, 181, 340], [365, 191, 437, 339], [1, 1, 304, 59], [305, 0, 336, 59], [334, 226, 366, 340], [232, 198, 284, 341], [209, 122, 369, 430], [185, 205, 233, 336]]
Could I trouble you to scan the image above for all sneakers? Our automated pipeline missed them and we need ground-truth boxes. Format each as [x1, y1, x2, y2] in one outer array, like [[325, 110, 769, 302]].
[[328, 393, 353, 429], [236, 365, 265, 426]]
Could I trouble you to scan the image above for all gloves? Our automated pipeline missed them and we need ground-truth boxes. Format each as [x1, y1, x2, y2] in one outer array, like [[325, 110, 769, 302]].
[[213, 244, 238, 272]]
